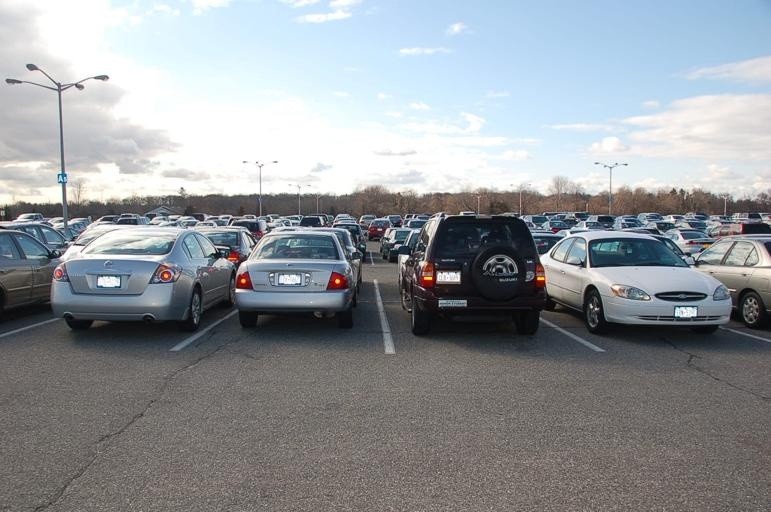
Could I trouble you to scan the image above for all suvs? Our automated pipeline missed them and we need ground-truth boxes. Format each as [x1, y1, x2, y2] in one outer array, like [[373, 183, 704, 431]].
[[393, 212, 549, 335]]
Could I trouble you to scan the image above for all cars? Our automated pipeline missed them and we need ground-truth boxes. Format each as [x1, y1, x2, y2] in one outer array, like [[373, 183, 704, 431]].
[[50, 227, 236, 329], [539, 229, 732, 334], [379, 212, 771, 264], [682, 236, 771, 331], [0, 211, 367, 271], [358, 214, 378, 230], [368, 218, 392, 241], [233, 230, 357, 331], [0, 230, 66, 316]]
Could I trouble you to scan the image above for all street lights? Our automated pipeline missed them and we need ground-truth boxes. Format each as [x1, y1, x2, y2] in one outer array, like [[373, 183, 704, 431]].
[[470, 159, 630, 214], [241, 159, 330, 219], [4, 62, 109, 227]]
